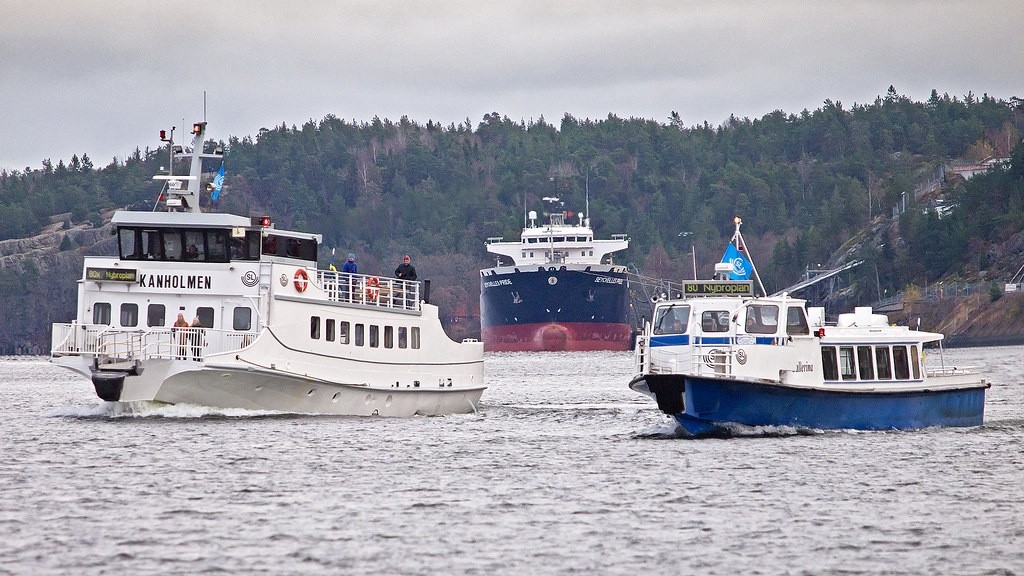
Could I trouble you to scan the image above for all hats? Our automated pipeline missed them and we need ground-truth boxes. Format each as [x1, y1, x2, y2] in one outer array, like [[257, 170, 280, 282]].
[[348, 253, 355, 258], [404, 255, 410, 260]]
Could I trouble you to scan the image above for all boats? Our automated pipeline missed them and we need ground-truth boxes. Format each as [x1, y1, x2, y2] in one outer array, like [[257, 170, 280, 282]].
[[630, 215, 990, 437], [477, 171, 635, 354], [48, 88, 487, 419]]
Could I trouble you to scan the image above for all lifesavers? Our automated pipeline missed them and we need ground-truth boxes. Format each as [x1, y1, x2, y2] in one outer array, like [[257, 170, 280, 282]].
[[294, 268, 308, 292], [368, 276, 379, 299]]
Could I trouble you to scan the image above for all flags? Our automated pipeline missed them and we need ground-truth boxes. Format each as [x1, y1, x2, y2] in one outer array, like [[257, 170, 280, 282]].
[[211, 160, 225, 202], [721, 243, 753, 280]]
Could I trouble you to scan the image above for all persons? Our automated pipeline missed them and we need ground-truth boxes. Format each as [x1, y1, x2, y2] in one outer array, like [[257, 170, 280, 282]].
[[747, 317, 755, 331], [392, 255, 417, 309], [172, 313, 203, 361], [673, 320, 682, 332], [343, 254, 358, 303], [186, 245, 200, 260]]
